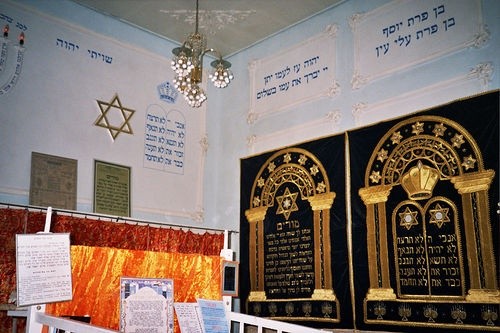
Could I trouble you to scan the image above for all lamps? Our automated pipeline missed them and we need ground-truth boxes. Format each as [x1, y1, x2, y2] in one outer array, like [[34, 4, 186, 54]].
[[170, 0, 233, 108]]
